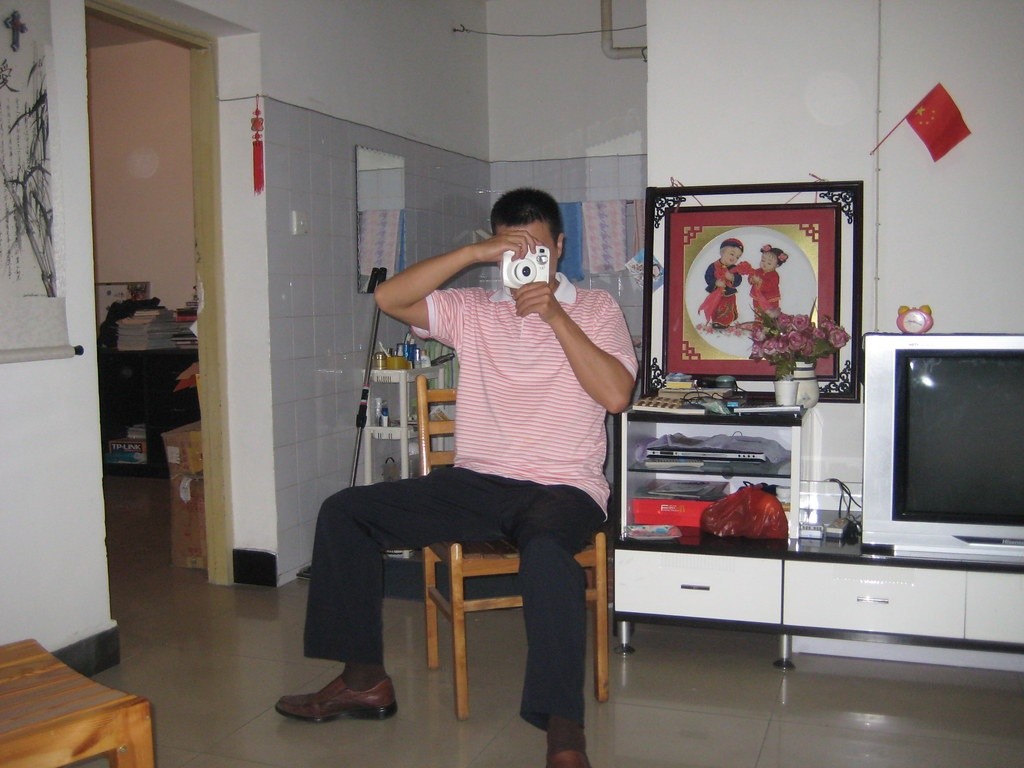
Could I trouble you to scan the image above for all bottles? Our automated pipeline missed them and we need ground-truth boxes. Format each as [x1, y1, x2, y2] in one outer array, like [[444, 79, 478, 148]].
[[375, 398, 390, 428], [374, 328, 455, 370]]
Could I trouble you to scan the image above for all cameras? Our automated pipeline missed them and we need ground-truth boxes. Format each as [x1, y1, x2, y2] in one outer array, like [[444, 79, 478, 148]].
[[500, 246, 550, 289]]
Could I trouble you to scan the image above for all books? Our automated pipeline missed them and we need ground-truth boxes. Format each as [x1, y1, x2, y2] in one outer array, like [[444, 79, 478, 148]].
[[117, 301, 198, 350]]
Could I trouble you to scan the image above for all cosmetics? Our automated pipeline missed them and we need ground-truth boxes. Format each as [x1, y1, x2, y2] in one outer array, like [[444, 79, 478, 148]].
[[375, 338, 431, 369]]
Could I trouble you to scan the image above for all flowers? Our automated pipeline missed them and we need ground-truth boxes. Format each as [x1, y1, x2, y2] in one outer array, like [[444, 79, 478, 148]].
[[732, 297, 852, 381]]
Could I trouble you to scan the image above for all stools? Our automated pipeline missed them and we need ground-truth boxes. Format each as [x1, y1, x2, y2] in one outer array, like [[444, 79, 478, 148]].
[[0, 637, 156, 768]]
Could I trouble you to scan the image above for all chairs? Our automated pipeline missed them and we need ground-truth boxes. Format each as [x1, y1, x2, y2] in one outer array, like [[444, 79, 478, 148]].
[[415, 374, 609, 721]]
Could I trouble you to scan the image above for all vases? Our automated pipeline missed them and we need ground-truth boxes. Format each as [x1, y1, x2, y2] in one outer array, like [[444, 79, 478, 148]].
[[772, 380, 800, 406], [781, 359, 819, 409]]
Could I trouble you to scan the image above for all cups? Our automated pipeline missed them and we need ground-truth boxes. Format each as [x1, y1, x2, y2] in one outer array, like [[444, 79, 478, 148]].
[[773, 381, 800, 406]]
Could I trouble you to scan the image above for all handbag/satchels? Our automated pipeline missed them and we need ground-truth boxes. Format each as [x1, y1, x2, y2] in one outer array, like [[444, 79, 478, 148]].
[[701, 485, 789, 539]]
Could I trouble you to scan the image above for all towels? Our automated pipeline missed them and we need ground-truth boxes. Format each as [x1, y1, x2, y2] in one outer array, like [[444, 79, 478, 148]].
[[632, 200, 645, 256], [582, 200, 628, 275], [400, 208, 405, 272], [556, 202, 584, 281], [359, 209, 398, 277]]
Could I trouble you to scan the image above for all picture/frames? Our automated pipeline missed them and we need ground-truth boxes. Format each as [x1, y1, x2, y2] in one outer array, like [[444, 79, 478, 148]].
[[637, 177, 864, 403]]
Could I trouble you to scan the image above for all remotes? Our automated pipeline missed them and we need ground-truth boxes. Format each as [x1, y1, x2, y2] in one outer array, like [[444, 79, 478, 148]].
[[825, 517, 850, 540], [733, 405, 805, 417], [645, 460, 704, 469]]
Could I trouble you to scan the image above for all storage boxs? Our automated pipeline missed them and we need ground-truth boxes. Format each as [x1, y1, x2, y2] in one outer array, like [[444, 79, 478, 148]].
[[631, 477, 732, 527], [108, 437, 148, 465], [160, 419, 209, 572]]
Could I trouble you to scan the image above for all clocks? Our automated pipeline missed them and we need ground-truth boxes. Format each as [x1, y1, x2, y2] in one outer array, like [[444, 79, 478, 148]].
[[896, 305, 934, 334]]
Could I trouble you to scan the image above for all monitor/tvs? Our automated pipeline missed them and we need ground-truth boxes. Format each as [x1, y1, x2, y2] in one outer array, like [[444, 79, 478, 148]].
[[861, 333, 1024, 556]]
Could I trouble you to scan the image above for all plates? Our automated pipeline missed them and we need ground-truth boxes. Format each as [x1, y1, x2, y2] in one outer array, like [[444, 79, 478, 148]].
[[626, 524, 683, 541]]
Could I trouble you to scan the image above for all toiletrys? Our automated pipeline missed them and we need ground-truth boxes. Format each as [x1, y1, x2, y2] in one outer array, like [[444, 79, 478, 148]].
[[374, 396, 390, 428], [372, 329, 455, 371]]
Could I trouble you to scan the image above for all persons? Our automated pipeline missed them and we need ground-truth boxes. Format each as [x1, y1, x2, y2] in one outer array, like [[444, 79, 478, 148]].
[[275, 187, 639, 768]]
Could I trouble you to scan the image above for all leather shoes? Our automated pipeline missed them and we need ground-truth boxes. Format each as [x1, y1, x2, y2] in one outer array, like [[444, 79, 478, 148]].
[[275, 675, 398, 721], [546, 747, 591, 768]]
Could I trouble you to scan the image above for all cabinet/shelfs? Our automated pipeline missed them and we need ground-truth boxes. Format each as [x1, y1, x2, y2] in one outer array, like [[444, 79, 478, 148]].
[[611, 542, 1024, 669], [97, 346, 201, 477], [360, 363, 445, 558]]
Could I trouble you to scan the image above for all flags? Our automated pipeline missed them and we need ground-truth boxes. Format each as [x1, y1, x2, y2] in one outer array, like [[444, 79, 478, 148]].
[[906, 83, 972, 163]]
